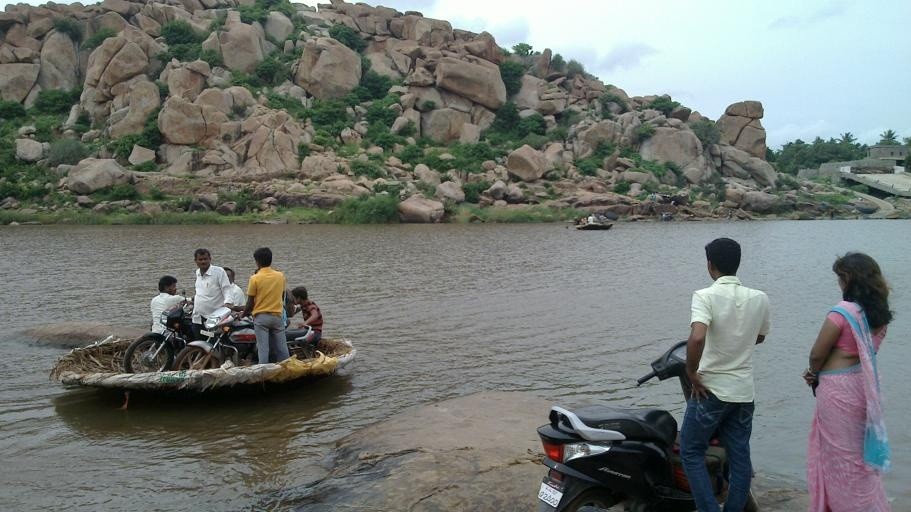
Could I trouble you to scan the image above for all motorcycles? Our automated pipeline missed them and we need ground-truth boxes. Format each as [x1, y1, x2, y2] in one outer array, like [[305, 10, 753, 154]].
[[168, 304, 317, 371], [122, 287, 255, 373], [532, 337, 764, 512]]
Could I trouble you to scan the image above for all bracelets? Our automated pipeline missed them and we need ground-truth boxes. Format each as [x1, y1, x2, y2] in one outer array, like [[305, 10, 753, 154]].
[[808, 368, 818, 376]]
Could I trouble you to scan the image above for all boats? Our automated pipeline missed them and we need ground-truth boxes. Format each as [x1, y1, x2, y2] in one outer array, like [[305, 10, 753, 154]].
[[45, 326, 363, 393], [574, 222, 614, 230]]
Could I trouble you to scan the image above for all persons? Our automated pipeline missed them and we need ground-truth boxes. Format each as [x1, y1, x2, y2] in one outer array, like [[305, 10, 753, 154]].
[[802, 251, 896, 512], [151, 246, 323, 365], [575, 213, 610, 225], [678, 236, 771, 511]]
